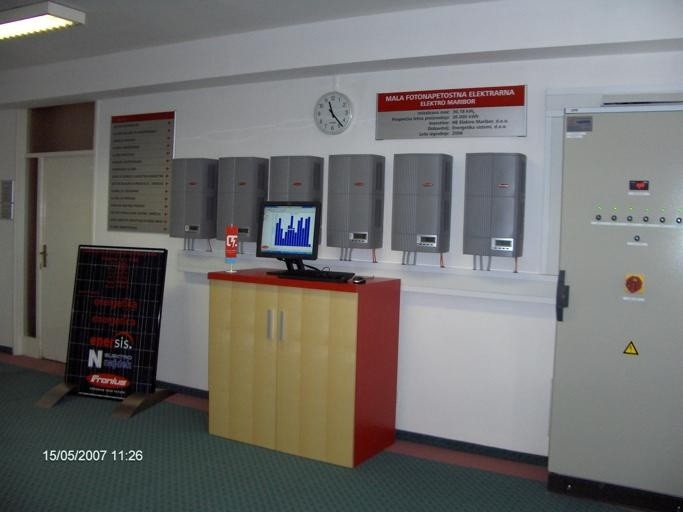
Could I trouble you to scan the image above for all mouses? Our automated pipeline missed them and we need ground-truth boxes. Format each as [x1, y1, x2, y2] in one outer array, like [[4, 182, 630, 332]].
[[353, 277, 365, 284]]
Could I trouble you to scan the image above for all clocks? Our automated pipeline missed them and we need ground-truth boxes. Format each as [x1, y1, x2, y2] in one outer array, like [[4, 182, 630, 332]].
[[313, 91, 353, 135]]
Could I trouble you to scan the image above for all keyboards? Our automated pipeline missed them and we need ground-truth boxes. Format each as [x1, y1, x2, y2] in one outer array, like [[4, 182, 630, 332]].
[[293, 270, 355, 283]]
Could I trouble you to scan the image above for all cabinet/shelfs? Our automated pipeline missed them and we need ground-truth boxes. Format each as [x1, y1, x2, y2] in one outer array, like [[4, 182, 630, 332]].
[[207, 266, 401, 469]]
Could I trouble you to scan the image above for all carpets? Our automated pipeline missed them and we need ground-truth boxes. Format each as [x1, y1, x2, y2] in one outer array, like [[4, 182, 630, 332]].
[[0, 361, 647, 512]]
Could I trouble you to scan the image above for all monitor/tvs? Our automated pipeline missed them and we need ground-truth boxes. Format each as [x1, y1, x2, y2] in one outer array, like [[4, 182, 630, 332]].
[[256, 201, 321, 275]]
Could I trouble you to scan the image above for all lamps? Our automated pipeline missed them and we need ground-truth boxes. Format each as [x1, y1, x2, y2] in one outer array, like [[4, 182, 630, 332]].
[[0, 1, 87, 42]]
